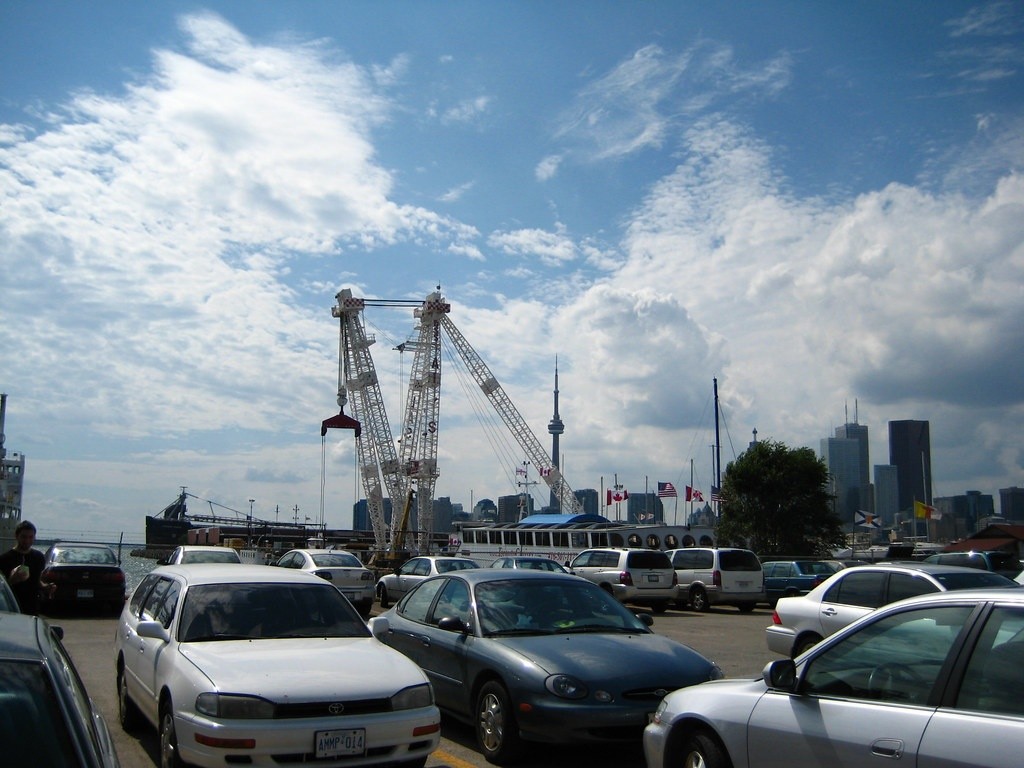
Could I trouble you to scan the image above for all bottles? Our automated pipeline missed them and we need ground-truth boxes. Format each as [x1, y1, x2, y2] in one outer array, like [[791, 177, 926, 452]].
[[49, 582, 55, 600]]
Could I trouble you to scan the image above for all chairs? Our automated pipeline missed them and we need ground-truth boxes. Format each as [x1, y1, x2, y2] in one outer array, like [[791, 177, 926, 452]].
[[530, 563, 542, 570], [87, 554, 102, 563], [446, 566, 457, 571], [966, 641, 1024, 715], [56, 551, 71, 563]]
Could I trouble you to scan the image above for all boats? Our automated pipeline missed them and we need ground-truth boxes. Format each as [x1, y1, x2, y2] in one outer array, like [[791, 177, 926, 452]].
[[144, 486, 463, 564], [0, 393, 25, 554]]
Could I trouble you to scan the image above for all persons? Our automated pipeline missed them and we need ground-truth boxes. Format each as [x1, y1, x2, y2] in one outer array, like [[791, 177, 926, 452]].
[[0, 520, 58, 617]]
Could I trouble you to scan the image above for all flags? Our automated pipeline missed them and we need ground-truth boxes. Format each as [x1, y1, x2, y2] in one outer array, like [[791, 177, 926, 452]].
[[687, 486, 704, 502], [712, 486, 727, 502], [647, 513, 654, 520], [915, 501, 942, 520], [516, 468, 527, 476], [450, 538, 459, 545], [640, 514, 645, 521], [855, 510, 882, 529], [607, 489, 629, 506], [540, 468, 550, 476], [658, 482, 677, 497]]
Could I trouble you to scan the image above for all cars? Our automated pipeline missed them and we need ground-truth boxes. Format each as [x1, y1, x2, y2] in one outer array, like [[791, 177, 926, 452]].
[[765, 564, 1024, 662], [0, 612, 122, 768], [375, 556, 481, 609], [487, 556, 568, 574], [733, 561, 841, 612], [921, 549, 1024, 581], [267, 548, 377, 618], [39, 542, 126, 615], [114, 563, 443, 768], [1012, 557, 1024, 588], [156, 545, 243, 564], [367, 567, 729, 765], [819, 548, 939, 578], [642, 587, 1024, 768]]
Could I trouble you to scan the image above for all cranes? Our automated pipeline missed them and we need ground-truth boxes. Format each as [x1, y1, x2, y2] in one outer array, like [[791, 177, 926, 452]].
[[321, 288, 587, 573]]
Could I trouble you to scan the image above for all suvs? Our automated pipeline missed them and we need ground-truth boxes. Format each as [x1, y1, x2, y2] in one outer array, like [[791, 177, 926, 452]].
[[660, 548, 767, 615], [552, 548, 681, 615]]
